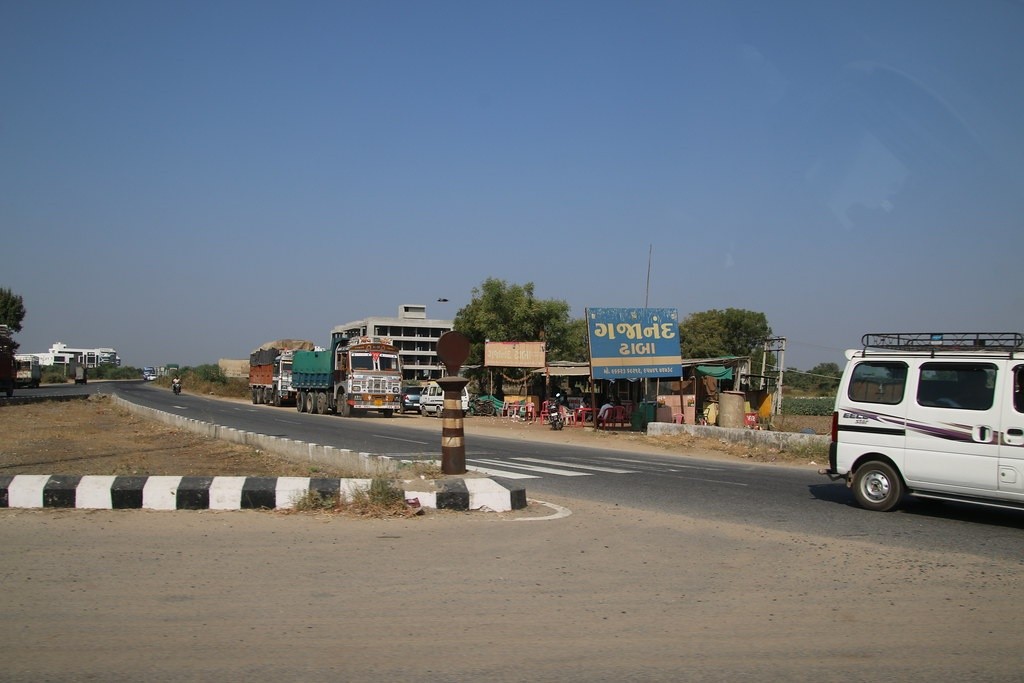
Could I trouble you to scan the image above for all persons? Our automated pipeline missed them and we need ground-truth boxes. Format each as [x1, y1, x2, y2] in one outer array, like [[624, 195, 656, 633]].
[[597, 399, 613, 429], [578, 397, 593, 422], [172, 375, 181, 389]]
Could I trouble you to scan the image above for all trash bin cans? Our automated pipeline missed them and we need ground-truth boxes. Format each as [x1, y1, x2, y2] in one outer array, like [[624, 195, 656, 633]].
[[718, 390, 745, 429], [631, 402, 656, 432]]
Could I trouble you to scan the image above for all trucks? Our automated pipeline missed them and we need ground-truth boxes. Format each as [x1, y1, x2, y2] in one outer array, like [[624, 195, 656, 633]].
[[142, 365, 157, 381], [0, 350, 22, 397], [11, 354, 44, 389]]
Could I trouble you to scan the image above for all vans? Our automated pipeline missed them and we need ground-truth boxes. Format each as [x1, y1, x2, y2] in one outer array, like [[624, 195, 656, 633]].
[[419, 383, 470, 417], [817, 332, 1024, 513]]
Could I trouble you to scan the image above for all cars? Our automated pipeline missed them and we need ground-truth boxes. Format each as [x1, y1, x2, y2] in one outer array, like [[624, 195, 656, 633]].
[[147, 373, 158, 382]]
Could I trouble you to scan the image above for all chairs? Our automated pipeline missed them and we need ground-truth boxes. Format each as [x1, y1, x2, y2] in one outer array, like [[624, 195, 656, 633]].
[[613, 406, 626, 428], [499, 400, 576, 428], [597, 408, 614, 430]]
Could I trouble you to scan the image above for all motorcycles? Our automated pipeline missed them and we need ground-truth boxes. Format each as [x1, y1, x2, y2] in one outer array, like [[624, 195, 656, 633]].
[[173, 379, 182, 396], [467, 393, 496, 417], [541, 393, 564, 431]]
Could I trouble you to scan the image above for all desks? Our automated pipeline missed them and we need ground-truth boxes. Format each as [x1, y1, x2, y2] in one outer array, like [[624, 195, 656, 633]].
[[575, 408, 599, 428], [508, 405, 535, 423]]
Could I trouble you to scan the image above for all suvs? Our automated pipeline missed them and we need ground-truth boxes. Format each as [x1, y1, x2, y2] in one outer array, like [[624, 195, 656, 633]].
[[393, 385, 423, 414]]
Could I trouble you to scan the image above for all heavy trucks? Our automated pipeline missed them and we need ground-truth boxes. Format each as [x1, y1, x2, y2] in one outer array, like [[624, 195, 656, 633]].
[[291, 335, 404, 417], [249, 348, 298, 407]]
